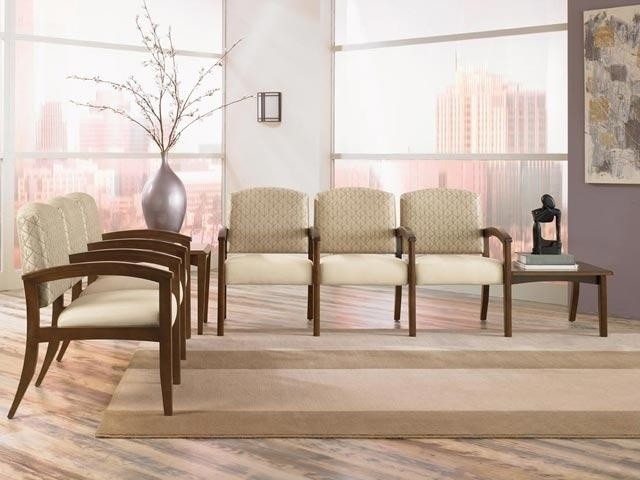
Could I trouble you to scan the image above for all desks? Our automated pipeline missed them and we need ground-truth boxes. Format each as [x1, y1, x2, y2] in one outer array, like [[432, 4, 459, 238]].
[[511, 262, 614, 337]]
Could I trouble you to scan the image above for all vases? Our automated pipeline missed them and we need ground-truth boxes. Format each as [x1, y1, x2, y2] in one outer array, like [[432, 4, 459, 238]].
[[141, 151, 187, 233]]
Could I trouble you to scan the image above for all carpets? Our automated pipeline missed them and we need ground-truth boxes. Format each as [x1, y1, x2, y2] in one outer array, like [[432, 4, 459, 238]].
[[95, 329, 639, 439]]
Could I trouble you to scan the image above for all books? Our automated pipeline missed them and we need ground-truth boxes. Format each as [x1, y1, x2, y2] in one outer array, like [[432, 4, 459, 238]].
[[516, 250, 577, 265], [513, 260, 578, 271]]
